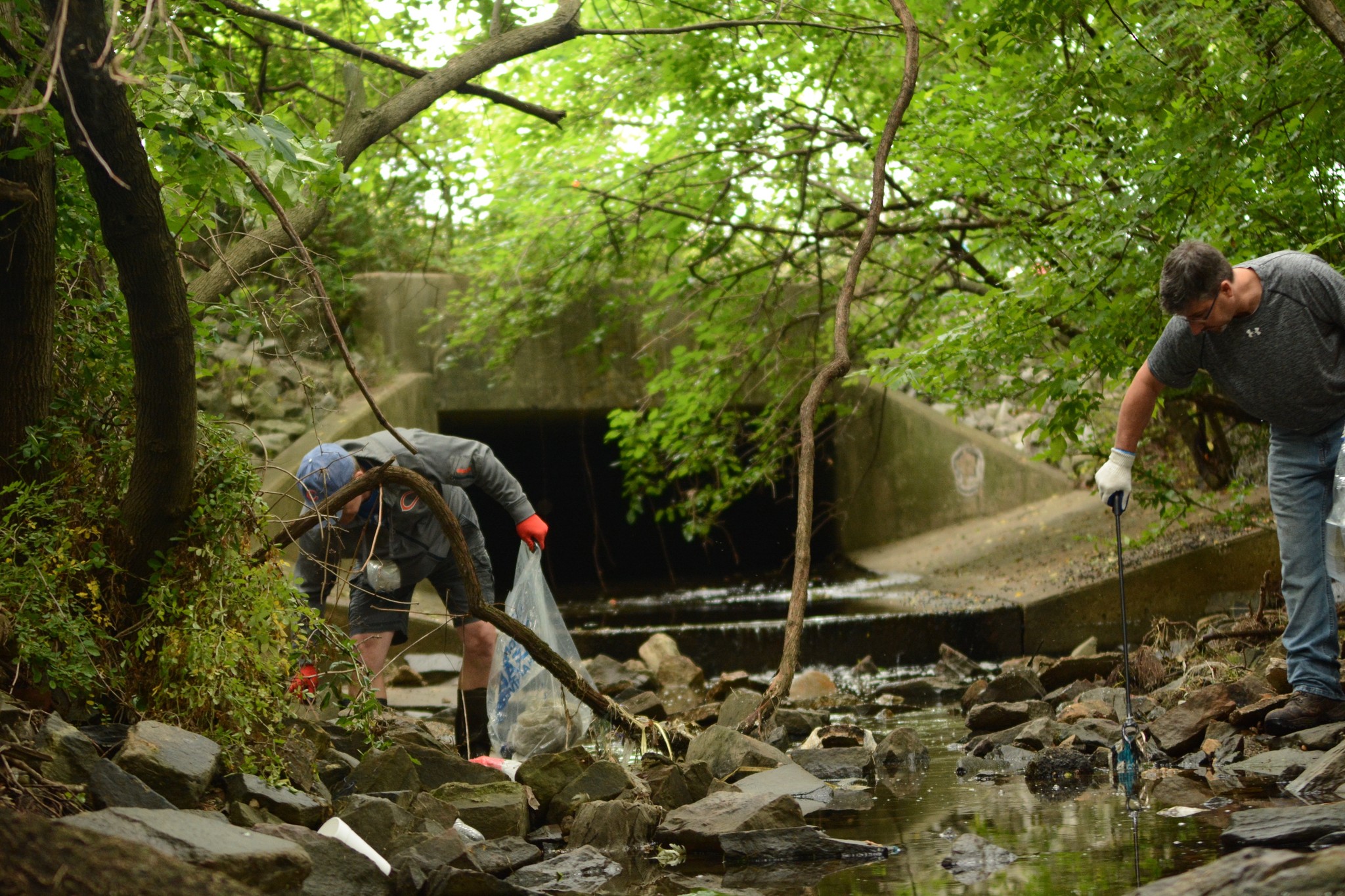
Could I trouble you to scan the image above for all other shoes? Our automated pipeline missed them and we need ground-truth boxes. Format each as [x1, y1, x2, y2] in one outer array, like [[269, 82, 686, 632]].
[[1265, 691, 1345, 737]]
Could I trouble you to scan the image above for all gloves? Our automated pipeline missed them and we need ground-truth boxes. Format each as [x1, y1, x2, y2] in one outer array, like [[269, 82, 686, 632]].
[[1095, 447, 1136, 517], [517, 514, 548, 550]]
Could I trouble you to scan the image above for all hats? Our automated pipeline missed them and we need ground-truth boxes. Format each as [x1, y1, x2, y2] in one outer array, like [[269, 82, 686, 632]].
[[296, 443, 355, 528]]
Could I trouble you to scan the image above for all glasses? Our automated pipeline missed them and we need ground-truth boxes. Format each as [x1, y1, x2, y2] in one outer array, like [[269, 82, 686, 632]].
[[1176, 289, 1220, 322]]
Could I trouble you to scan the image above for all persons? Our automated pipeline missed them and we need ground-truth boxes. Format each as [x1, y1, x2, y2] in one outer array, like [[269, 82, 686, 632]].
[[284, 421, 545, 765], [1092, 238, 1345, 729]]
